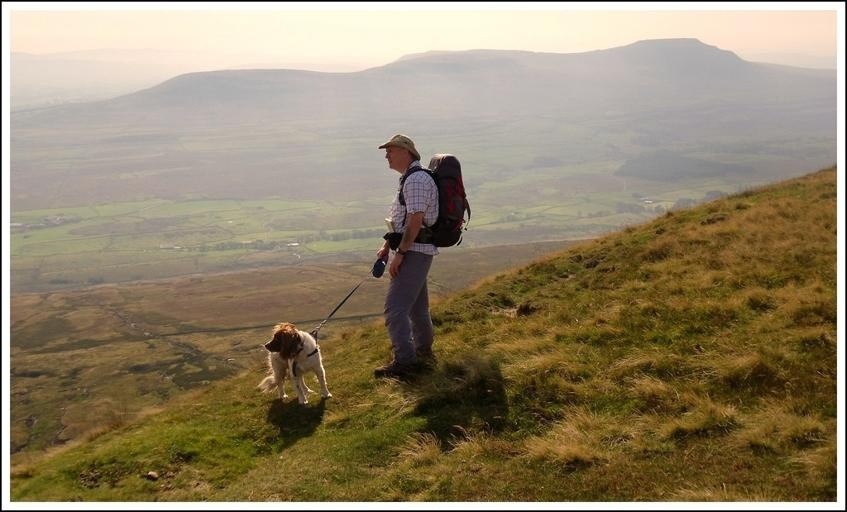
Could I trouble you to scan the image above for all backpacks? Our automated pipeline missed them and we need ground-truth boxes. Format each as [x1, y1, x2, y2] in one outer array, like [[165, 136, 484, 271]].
[[399, 154, 471, 247]]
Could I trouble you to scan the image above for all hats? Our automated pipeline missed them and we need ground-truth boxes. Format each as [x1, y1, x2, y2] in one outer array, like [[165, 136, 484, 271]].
[[378, 135, 420, 161]]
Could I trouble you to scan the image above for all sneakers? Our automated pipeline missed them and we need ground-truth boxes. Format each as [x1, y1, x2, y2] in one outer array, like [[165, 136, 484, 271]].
[[375, 347, 432, 378]]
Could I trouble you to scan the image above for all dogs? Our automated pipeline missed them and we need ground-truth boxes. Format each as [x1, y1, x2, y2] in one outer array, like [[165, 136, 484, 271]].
[[257, 321, 333, 405]]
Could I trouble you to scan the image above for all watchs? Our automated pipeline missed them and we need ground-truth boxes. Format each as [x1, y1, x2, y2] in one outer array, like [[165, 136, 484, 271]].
[[396, 247, 406, 256]]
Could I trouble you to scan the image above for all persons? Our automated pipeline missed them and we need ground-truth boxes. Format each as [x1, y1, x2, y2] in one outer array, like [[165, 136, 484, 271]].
[[376, 135, 439, 377]]
